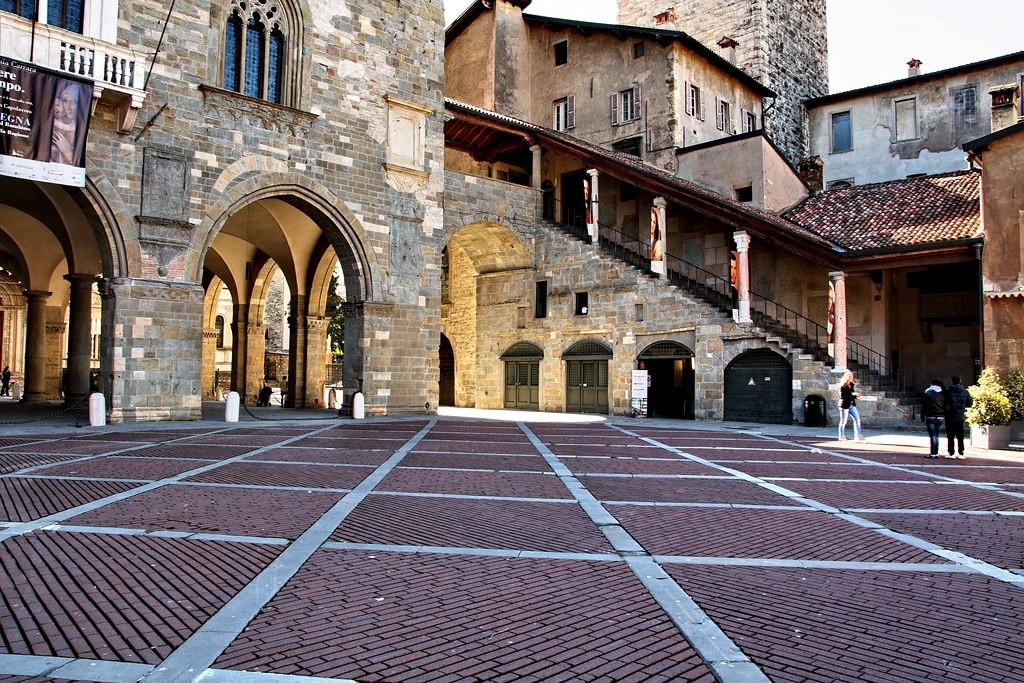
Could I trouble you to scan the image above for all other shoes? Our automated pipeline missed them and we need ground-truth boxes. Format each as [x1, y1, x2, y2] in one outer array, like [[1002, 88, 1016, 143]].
[[957, 455, 966, 460], [854, 435, 865, 440], [839, 435, 847, 441], [945, 454, 956, 459], [924, 453, 939, 459]]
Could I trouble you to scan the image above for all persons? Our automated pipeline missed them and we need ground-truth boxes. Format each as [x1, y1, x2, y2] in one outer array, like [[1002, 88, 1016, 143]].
[[919, 375, 973, 460], [837, 372, 865, 440], [50, 84, 80, 165], [731, 252, 739, 311], [0, 365, 12, 398], [257, 376, 289, 408], [584, 179, 592, 225], [827, 281, 835, 344], [651, 208, 663, 261]]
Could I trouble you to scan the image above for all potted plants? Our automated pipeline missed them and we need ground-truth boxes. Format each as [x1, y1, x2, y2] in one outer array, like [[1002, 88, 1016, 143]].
[[963, 365, 1024, 449]]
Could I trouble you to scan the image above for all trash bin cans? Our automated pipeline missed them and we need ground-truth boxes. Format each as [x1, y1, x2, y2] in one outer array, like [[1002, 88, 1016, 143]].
[[803, 393, 827, 428]]
